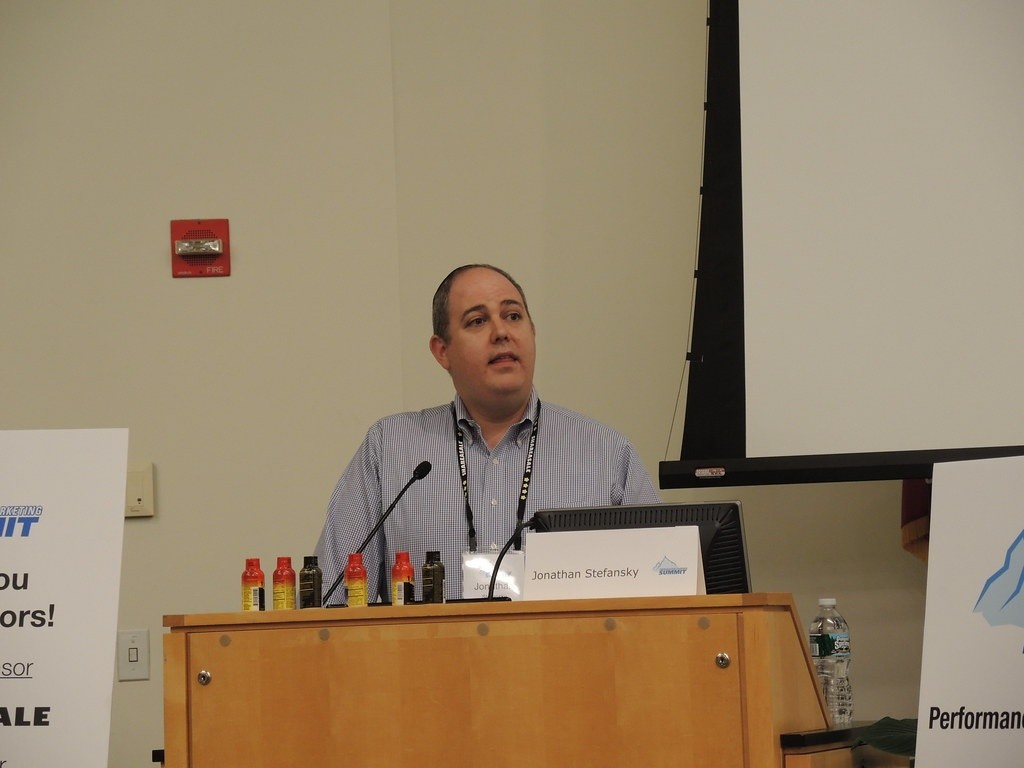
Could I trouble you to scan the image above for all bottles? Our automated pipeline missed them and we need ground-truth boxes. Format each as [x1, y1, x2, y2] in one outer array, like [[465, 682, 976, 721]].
[[272, 557, 296, 612], [393, 552, 415, 606], [242, 558, 266, 612], [809, 598, 855, 724], [421, 550, 447, 604], [299, 556, 321, 610], [342, 553, 368, 608]]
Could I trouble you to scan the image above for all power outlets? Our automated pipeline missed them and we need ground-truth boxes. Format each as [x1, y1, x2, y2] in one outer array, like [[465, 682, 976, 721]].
[[123, 460, 153, 518]]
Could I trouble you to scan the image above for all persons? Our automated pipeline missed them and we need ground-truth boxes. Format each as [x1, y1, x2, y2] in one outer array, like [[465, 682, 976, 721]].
[[297, 265, 664, 606]]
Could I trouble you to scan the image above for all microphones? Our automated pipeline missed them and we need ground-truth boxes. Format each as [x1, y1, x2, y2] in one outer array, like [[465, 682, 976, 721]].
[[319, 461, 431, 606]]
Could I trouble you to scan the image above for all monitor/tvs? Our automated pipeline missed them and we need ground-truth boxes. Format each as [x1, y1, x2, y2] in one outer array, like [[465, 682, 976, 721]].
[[534, 500, 752, 594]]
